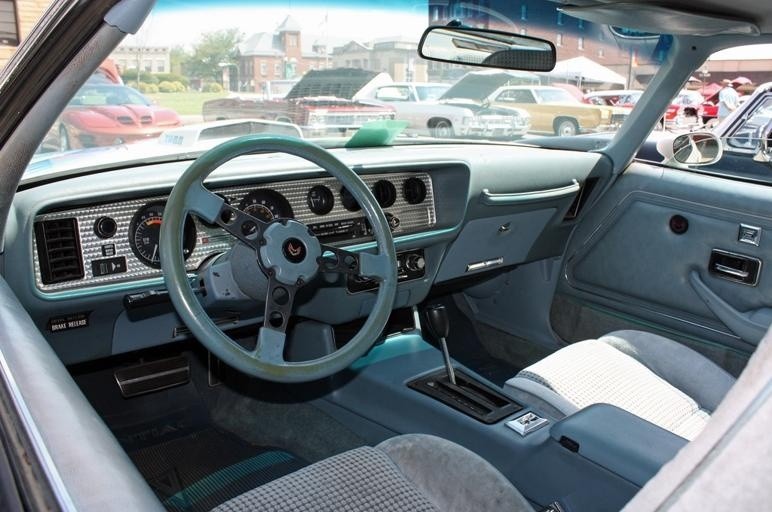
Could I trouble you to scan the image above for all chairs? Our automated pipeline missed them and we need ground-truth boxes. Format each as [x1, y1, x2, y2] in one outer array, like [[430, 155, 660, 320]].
[[210, 322, 772, 511], [505, 327, 772, 423]]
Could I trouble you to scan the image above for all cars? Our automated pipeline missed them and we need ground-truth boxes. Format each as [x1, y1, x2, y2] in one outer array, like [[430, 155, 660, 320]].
[[35, 83, 183, 155]]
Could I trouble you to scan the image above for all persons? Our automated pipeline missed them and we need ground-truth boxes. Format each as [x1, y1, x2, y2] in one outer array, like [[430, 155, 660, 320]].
[[718, 79, 742, 126]]
